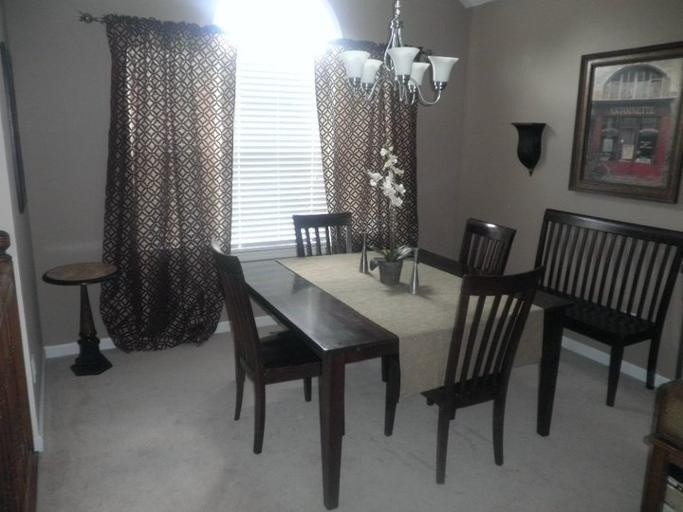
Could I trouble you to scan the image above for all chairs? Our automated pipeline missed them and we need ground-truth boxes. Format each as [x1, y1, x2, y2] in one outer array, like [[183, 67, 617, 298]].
[[461, 217, 515, 276], [382, 265, 546, 482], [211, 237, 395, 454], [292, 209, 352, 256]]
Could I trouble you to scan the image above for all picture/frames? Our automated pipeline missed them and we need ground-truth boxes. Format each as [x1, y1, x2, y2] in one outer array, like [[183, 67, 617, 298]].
[[567, 39, 682, 204]]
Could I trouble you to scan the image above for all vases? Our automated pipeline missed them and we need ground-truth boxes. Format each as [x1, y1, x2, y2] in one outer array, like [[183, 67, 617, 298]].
[[378, 262, 403, 286]]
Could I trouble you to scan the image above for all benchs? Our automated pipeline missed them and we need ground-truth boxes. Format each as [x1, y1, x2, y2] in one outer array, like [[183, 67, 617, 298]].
[[534, 207, 681, 407]]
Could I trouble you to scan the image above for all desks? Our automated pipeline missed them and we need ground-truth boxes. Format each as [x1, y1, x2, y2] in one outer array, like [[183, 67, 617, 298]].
[[41, 260, 124, 377]]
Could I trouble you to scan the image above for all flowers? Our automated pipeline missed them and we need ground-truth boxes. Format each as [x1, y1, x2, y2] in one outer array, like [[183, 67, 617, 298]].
[[362, 130, 420, 271]]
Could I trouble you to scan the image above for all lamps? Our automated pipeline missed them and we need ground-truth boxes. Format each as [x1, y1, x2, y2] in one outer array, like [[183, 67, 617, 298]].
[[338, 1, 460, 109]]
